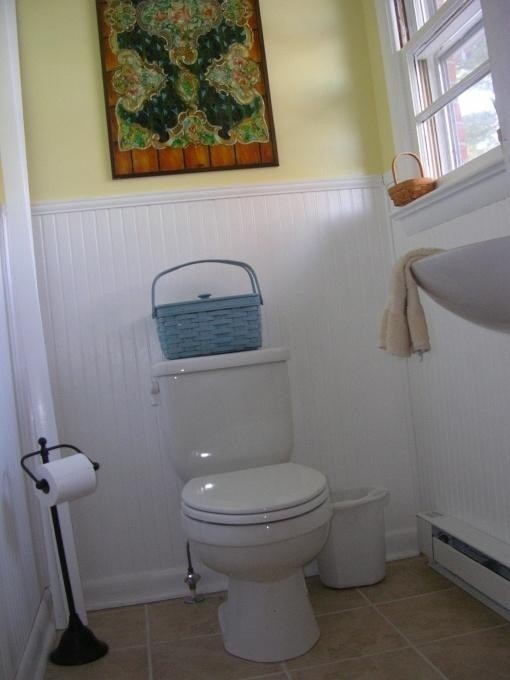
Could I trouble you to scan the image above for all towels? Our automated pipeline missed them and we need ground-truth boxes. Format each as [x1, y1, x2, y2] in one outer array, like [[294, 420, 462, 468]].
[[375, 245, 450, 362]]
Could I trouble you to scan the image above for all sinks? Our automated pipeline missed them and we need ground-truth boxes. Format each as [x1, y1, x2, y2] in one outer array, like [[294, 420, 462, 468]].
[[410, 236, 510, 334]]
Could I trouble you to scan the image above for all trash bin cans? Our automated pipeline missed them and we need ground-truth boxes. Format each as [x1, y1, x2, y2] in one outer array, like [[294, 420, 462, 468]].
[[317, 485, 389, 589]]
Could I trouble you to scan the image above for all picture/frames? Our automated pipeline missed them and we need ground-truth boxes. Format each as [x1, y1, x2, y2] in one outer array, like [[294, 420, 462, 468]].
[[96, 0, 281, 179]]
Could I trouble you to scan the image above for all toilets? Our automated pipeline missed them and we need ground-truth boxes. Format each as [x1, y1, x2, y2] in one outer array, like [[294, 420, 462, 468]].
[[149, 346, 333, 661]]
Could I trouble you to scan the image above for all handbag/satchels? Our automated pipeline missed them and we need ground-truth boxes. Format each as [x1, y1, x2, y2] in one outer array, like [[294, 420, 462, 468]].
[[151, 257, 265, 359]]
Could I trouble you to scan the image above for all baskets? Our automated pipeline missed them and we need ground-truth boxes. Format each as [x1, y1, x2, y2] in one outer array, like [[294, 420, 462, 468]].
[[388, 153, 434, 206]]
